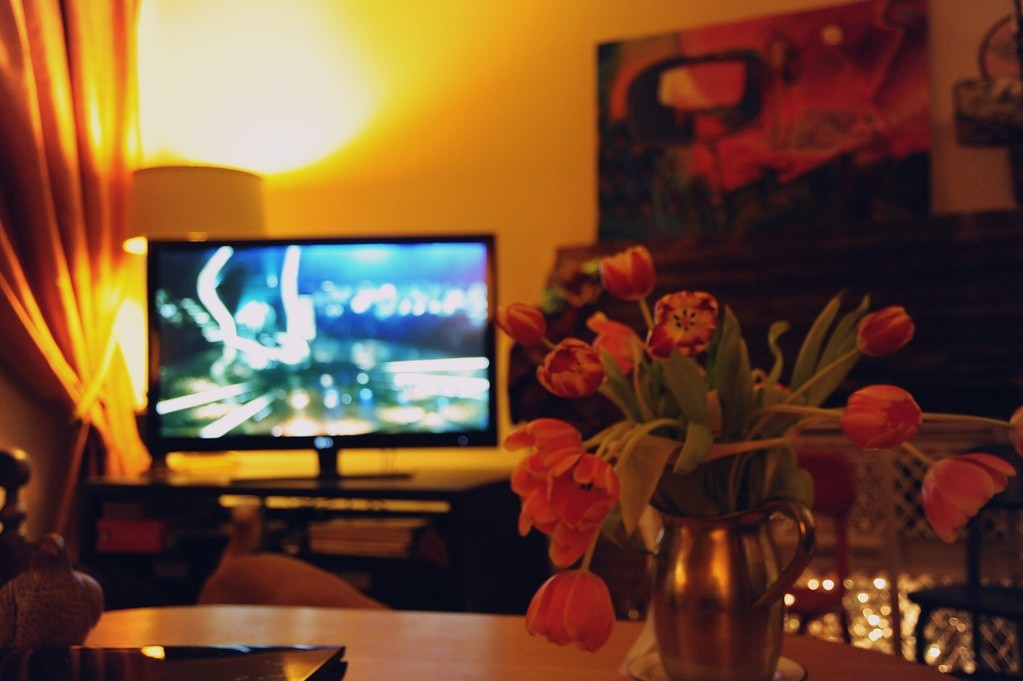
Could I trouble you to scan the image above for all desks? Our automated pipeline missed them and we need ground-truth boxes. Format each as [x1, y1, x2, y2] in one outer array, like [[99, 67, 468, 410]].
[[80, 469, 548, 610], [82, 604, 952, 681]]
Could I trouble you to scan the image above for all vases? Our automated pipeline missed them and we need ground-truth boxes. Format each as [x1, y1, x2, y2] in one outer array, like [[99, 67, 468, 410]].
[[654, 496, 819, 681]]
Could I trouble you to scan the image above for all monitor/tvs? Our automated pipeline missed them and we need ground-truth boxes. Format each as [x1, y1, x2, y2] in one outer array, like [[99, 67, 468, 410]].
[[146, 232, 498, 482]]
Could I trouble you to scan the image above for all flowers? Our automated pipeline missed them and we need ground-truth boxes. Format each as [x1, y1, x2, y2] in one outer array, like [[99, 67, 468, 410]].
[[464, 253, 1023, 654]]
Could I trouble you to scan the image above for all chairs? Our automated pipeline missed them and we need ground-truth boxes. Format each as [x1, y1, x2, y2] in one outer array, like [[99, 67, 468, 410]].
[[908, 440, 1023, 681], [763, 449, 855, 646]]
